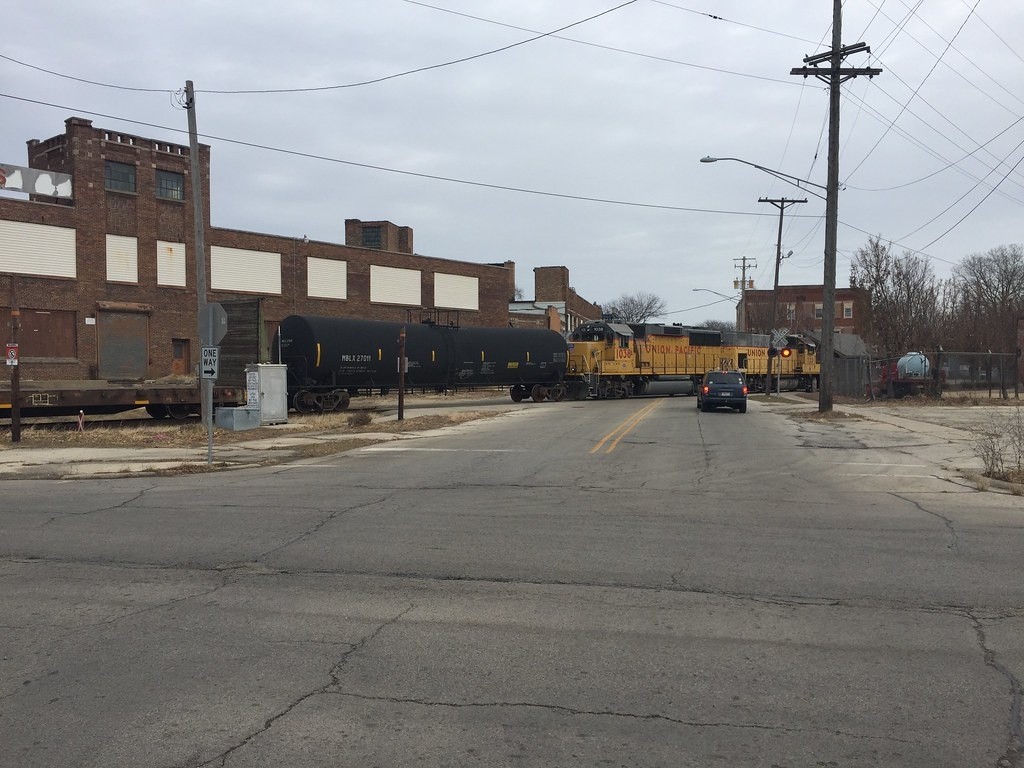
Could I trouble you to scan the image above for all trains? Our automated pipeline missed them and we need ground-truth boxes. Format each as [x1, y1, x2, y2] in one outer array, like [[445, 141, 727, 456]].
[[271, 312, 820, 414]]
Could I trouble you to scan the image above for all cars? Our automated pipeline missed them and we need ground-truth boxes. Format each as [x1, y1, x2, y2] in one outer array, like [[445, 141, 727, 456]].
[[697, 371, 748, 414]]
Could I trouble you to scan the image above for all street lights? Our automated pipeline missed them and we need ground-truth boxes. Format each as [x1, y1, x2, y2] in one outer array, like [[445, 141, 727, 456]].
[[700, 156, 840, 412], [692, 288, 745, 331]]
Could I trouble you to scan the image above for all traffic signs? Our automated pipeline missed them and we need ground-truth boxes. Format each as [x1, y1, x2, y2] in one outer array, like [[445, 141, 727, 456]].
[[201, 346, 220, 380]]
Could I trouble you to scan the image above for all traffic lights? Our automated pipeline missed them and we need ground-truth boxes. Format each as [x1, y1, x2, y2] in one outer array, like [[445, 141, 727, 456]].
[[781, 348, 792, 357]]
